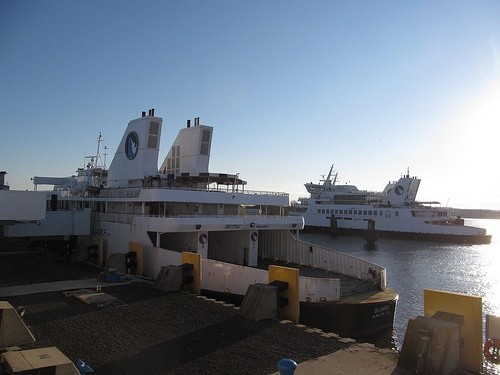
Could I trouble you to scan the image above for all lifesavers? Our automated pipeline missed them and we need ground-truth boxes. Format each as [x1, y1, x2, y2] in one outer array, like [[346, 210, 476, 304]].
[[484, 338, 500, 364]]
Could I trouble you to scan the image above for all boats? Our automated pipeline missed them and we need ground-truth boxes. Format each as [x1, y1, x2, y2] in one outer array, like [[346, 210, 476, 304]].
[[287, 159, 492, 244], [0, 108, 398, 336]]
